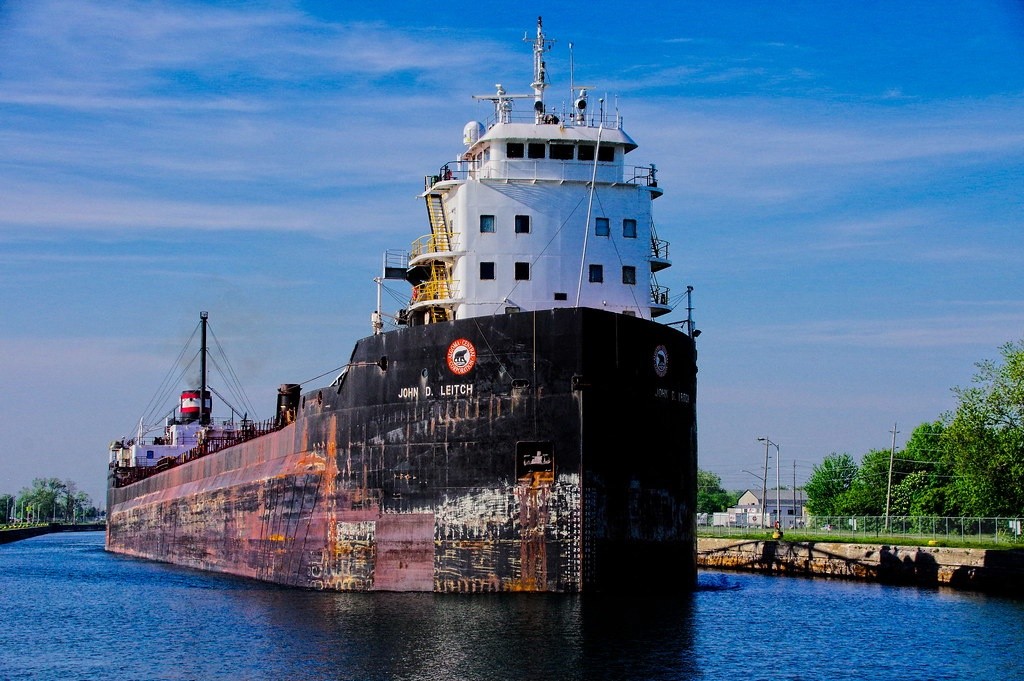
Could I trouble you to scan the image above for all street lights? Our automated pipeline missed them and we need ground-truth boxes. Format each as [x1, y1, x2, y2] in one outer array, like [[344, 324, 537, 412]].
[[740, 468, 765, 529], [758, 437, 781, 528], [6, 493, 40, 524]]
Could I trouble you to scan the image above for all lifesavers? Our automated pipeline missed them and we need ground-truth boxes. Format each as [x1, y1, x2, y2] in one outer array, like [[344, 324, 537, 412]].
[[413, 289, 418, 298]]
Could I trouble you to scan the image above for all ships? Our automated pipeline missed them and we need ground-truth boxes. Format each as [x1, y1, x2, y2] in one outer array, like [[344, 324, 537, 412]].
[[102, 14, 703, 598]]
